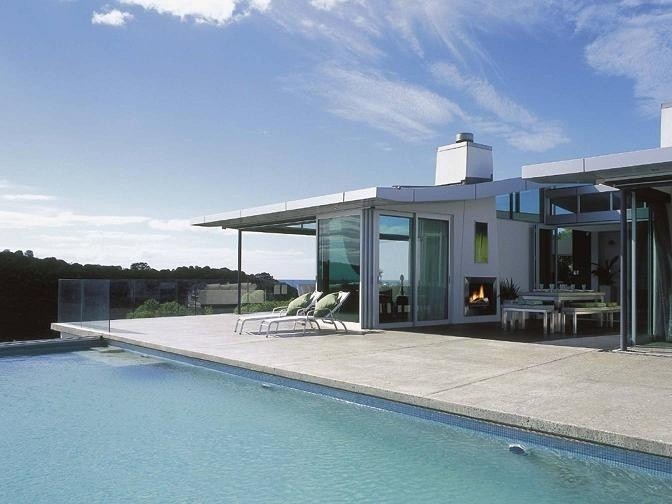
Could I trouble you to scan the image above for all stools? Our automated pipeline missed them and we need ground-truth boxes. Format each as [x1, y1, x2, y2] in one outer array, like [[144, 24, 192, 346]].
[[394, 295, 409, 319]]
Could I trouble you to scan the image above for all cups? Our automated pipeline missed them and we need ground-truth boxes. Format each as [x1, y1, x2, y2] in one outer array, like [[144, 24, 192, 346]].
[[538, 284, 587, 289]]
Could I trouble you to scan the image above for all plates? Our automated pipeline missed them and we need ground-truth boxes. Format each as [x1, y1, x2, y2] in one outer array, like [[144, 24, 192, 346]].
[[577, 289, 584, 292], [585, 290, 595, 292], [564, 290, 573, 292]]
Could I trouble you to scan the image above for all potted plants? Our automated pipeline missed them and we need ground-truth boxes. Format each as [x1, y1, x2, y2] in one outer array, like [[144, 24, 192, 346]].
[[500, 279, 521, 303], [590, 254, 620, 302]]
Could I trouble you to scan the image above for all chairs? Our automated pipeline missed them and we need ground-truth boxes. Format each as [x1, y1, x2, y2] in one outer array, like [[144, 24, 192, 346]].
[[234, 289, 351, 338]]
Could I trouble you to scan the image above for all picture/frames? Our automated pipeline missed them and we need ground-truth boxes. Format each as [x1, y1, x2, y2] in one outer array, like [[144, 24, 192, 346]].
[[473, 222, 489, 264]]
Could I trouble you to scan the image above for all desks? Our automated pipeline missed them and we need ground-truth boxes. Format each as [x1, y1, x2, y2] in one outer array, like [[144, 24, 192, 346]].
[[379, 285, 393, 318]]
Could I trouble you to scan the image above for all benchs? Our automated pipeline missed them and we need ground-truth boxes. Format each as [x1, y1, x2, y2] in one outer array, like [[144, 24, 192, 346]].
[[502, 303, 621, 336]]
